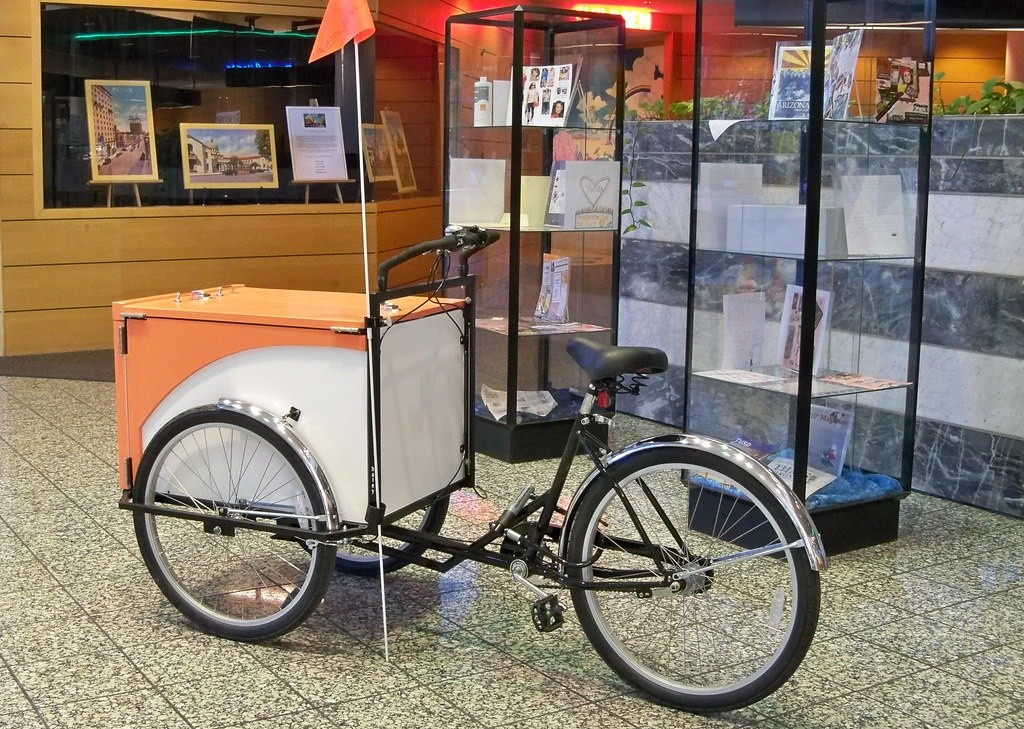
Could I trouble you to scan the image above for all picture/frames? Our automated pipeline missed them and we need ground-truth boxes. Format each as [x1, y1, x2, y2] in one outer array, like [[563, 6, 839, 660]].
[[84, 80, 419, 194], [775, 284, 836, 377]]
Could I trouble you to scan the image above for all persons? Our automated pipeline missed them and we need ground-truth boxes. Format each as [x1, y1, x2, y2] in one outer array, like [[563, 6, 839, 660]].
[[523, 67, 569, 124]]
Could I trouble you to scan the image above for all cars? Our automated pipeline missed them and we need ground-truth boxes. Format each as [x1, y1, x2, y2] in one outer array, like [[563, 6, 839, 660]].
[[103, 157, 111, 165], [116, 152, 122, 157]]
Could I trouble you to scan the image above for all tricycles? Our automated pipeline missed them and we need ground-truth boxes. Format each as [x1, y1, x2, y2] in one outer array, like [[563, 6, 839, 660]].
[[103, 228, 829, 717]]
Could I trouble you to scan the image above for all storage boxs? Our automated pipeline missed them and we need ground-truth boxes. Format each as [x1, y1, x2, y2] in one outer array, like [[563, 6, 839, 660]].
[[110, 281, 466, 525]]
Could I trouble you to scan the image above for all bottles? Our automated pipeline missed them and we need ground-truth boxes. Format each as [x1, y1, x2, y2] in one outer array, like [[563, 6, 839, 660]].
[[474, 76, 493, 127]]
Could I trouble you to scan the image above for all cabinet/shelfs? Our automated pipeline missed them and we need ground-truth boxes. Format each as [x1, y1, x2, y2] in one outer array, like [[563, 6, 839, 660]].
[[440, 6, 627, 465], [680, 0, 935, 562]]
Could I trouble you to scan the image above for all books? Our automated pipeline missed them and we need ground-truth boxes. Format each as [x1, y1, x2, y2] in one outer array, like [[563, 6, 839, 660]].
[[807, 405, 855, 476], [534, 253, 571, 324], [695, 433, 839, 508], [776, 284, 835, 376], [544, 158, 566, 228]]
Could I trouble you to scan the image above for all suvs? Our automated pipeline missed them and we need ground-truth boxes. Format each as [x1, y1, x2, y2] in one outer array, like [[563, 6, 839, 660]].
[[221, 170, 238, 175], [140, 153, 145, 161]]
